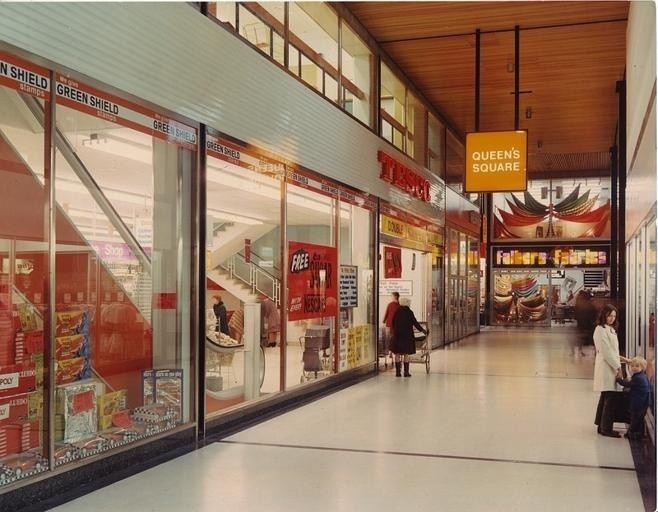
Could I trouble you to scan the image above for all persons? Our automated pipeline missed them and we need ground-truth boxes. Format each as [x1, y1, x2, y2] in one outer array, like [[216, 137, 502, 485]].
[[382, 291, 427, 378], [211, 295, 229, 336], [593, 303, 623, 438], [564, 288, 597, 357], [594, 355, 650, 432], [260, 295, 281, 347]]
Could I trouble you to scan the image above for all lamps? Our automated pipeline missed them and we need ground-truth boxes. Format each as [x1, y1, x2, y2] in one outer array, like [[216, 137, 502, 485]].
[[538, 141, 541, 148], [507, 64, 514, 72], [526, 109, 532, 118]]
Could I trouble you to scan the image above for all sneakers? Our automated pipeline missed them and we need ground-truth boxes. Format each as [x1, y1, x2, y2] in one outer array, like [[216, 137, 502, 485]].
[[598, 427, 621, 438]]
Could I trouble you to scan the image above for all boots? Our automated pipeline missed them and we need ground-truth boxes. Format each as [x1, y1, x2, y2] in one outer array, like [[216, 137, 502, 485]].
[[396, 362, 402, 377], [404, 362, 411, 377]]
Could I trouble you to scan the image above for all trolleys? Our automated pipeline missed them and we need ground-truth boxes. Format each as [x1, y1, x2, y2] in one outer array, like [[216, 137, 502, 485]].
[[400, 322, 430, 372]]
